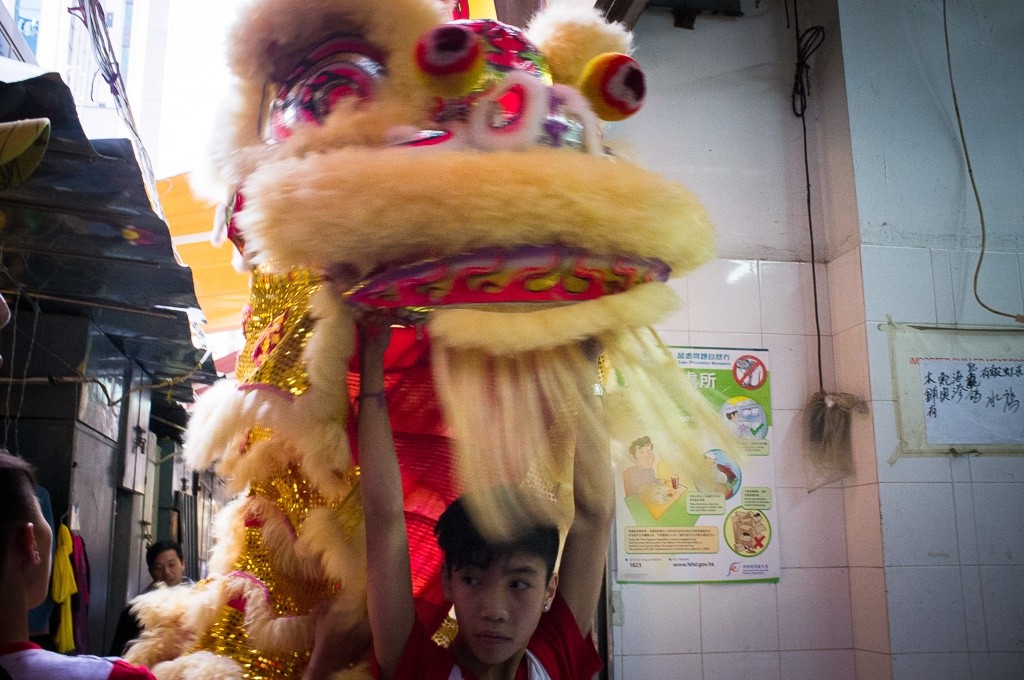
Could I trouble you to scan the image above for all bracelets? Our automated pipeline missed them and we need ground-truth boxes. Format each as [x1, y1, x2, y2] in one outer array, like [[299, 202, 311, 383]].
[[353, 393, 384, 400]]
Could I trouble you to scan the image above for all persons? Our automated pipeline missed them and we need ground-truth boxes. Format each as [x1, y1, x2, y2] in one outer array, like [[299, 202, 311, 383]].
[[292, 309, 616, 680], [0, 448, 158, 679], [108, 538, 198, 657]]
